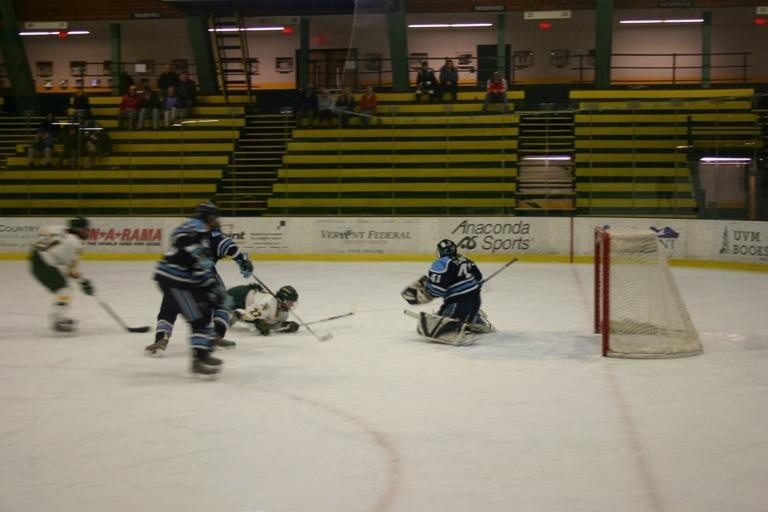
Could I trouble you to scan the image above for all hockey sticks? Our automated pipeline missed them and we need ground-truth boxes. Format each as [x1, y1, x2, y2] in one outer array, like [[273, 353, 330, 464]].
[[252, 272, 332, 342], [91, 293, 151, 332]]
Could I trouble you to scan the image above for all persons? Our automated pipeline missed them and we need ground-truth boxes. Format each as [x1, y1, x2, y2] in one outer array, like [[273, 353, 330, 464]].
[[30, 217, 94, 332], [25, 63, 198, 169], [295, 83, 377, 129], [483, 72, 511, 114], [415, 61, 438, 104], [401, 239, 496, 346], [437, 60, 458, 102], [144, 220, 253, 352], [227, 284, 299, 335], [151, 202, 222, 375]]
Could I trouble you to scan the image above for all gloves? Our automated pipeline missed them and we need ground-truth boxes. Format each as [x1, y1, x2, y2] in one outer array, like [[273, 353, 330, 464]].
[[239, 259, 253, 277], [81, 281, 92, 295], [193, 248, 214, 268]]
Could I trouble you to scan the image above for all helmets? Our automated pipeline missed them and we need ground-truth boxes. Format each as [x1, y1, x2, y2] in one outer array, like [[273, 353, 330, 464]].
[[195, 203, 218, 221], [69, 219, 89, 239], [438, 240, 456, 257], [277, 286, 298, 304]]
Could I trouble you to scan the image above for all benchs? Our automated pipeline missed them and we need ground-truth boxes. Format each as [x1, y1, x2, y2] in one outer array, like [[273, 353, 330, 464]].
[[0, 95, 255, 215], [267, 89, 527, 219], [569, 86, 762, 219]]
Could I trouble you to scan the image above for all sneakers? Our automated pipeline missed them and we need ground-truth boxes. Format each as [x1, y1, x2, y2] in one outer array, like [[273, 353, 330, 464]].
[[53, 318, 77, 331], [145, 336, 235, 364]]
